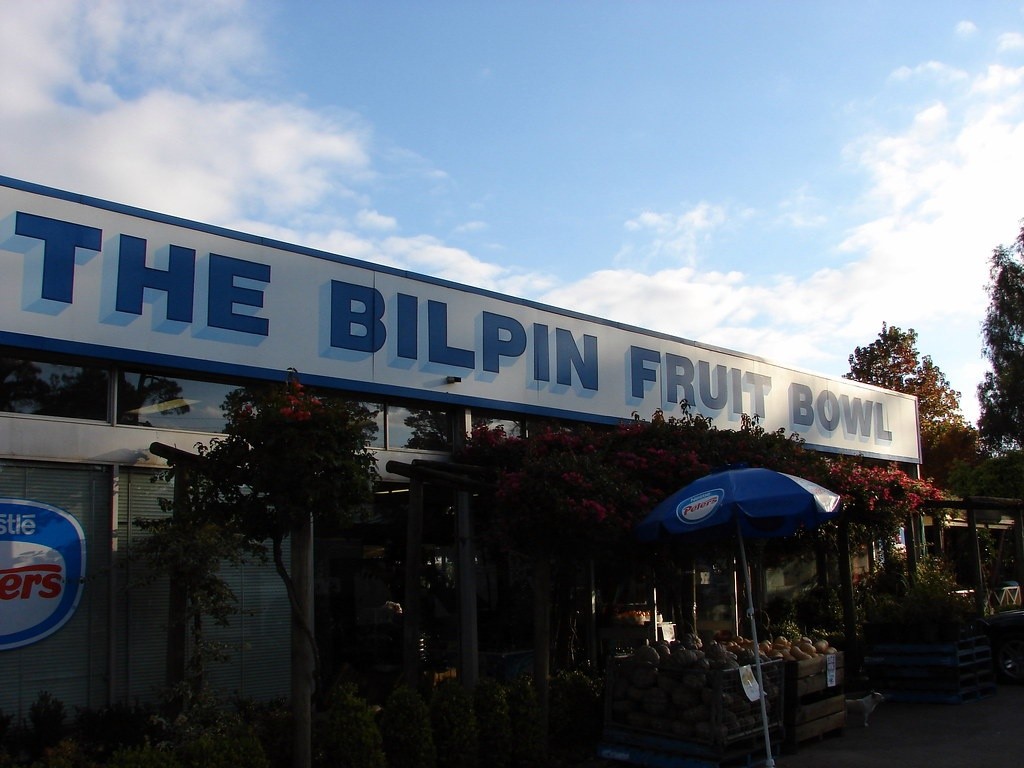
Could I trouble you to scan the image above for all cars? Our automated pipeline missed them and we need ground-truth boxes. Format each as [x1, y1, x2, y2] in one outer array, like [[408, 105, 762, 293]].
[[972, 608, 1024, 685]]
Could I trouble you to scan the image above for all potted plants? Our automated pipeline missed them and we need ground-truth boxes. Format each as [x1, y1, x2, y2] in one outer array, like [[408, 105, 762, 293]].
[[853, 544, 978, 645]]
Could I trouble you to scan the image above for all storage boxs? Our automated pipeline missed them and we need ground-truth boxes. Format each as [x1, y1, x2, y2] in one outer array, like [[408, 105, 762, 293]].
[[845, 693, 877, 728]]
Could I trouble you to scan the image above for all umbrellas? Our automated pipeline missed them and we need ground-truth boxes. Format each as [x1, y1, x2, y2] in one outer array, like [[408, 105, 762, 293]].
[[632, 463, 850, 768]]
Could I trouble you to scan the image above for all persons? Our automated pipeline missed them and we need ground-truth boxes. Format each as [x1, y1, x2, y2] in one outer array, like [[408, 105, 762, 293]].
[[943, 557, 1021, 607]]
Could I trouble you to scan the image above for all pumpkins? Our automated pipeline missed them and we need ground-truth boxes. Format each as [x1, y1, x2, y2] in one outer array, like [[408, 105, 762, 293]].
[[607, 632, 840, 742]]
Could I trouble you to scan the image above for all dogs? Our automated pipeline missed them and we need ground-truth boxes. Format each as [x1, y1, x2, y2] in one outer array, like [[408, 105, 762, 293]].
[[845, 689, 886, 728]]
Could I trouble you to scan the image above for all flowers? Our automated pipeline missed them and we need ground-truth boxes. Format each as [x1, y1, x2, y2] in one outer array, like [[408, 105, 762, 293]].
[[450, 412, 940, 566], [188, 367, 389, 537]]
